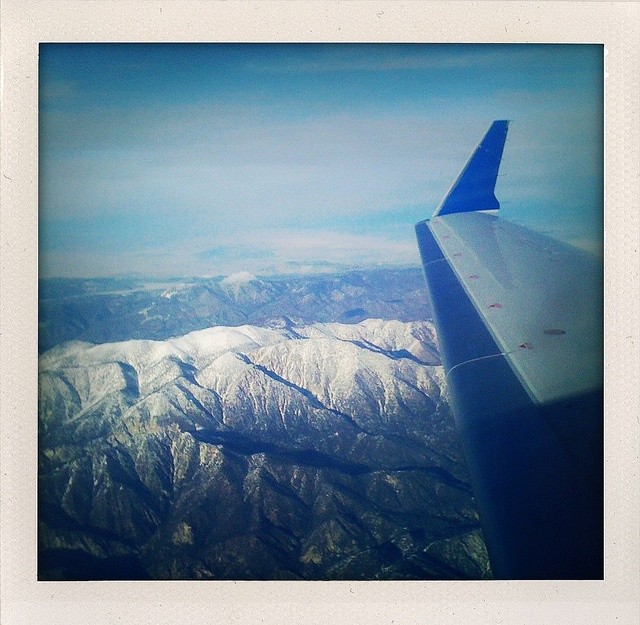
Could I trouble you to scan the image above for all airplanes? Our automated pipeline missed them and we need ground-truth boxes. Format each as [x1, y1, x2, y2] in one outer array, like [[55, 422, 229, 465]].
[[415, 119, 604, 580]]
